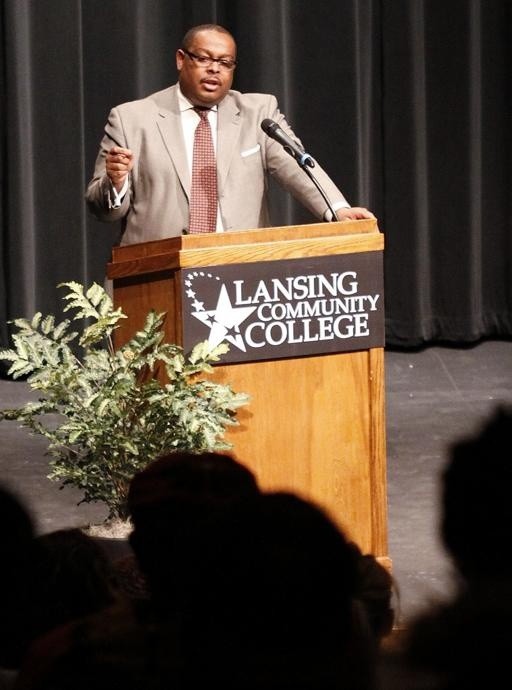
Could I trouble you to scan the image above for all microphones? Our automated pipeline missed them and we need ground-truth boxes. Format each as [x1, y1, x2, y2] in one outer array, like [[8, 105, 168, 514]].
[[261, 119, 315, 168]]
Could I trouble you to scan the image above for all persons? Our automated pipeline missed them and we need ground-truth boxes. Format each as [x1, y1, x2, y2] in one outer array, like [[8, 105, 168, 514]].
[[84, 25, 379, 249]]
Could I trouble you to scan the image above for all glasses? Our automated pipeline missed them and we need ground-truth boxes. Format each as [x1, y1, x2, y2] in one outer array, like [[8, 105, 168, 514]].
[[182, 48, 238, 67]]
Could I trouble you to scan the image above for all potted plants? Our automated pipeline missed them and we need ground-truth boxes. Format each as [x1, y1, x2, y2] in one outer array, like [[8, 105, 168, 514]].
[[0, 280, 252, 561]]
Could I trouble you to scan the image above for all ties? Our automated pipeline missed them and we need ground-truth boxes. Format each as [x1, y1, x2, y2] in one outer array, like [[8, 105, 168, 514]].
[[189, 106, 217, 234]]
